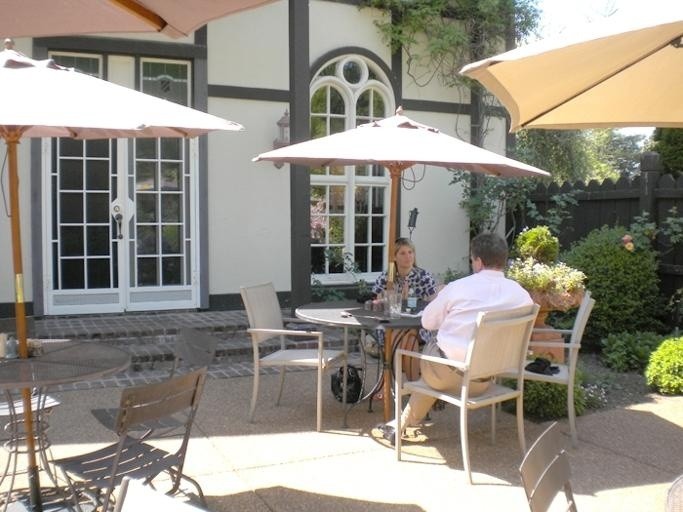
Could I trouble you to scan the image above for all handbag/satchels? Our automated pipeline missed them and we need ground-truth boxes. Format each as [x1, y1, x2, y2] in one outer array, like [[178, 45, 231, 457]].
[[331, 364, 363, 403]]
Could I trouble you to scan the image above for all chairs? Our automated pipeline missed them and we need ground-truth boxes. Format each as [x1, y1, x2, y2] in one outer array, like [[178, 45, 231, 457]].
[[517, 421, 578, 512], [240, 281, 349, 432], [49, 364, 208, 512], [394, 303, 541, 486], [497, 290, 599, 448], [90, 327, 218, 442]]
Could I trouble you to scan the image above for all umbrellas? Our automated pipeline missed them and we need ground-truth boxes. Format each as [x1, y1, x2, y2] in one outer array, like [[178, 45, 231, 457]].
[[0, 35, 243, 510], [456, 11, 681, 135], [252, 104, 553, 426]]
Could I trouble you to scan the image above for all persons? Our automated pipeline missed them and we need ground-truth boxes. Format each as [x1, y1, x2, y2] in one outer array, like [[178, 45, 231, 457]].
[[377, 232, 539, 442], [368, 237, 439, 392]]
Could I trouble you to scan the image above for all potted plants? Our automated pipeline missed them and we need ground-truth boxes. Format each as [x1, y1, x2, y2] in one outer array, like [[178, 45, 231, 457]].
[[318, 246, 377, 304]]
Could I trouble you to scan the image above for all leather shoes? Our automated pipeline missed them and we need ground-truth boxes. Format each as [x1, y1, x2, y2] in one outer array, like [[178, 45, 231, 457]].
[[377, 423, 395, 443]]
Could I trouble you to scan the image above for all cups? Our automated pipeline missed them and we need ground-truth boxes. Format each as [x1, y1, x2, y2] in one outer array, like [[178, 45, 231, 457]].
[[389, 294, 402, 319]]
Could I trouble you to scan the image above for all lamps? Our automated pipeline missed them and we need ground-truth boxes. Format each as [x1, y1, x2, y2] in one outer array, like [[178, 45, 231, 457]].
[[273, 107, 290, 170]]
